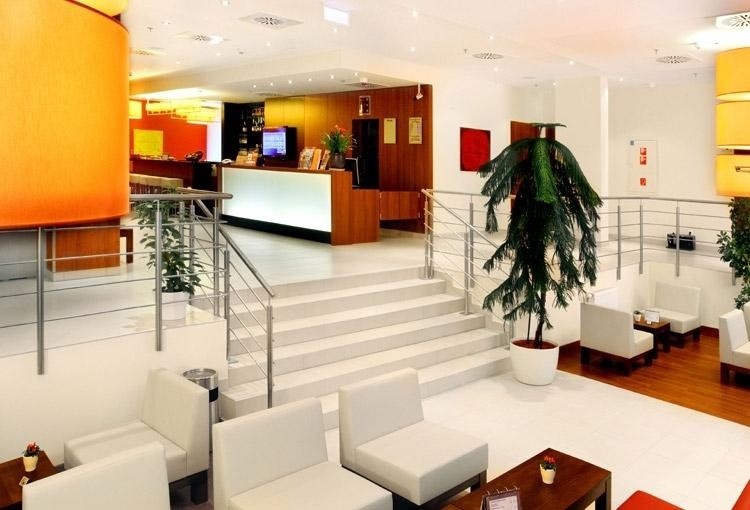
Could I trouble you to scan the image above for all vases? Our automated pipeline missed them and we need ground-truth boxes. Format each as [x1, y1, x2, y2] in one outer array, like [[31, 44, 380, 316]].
[[22, 454, 40, 473], [540, 465, 556, 484]]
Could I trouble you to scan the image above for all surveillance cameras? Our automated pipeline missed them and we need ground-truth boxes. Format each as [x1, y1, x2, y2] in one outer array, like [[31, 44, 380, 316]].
[[415, 93, 423, 100]]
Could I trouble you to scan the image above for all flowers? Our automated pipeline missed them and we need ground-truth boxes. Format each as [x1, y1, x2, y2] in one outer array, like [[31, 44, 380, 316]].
[[541, 454, 557, 471], [21, 443, 43, 460]]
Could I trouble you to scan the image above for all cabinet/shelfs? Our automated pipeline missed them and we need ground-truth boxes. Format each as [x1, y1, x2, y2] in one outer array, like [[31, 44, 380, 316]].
[[235, 103, 264, 159]]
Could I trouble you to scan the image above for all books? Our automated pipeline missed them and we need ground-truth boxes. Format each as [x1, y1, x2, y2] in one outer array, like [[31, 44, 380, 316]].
[[299, 147, 331, 170], [480, 487, 523, 510]]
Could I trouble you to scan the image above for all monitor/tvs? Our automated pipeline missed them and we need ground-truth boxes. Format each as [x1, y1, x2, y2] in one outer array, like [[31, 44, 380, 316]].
[[261, 126, 288, 160], [344, 158, 361, 188]]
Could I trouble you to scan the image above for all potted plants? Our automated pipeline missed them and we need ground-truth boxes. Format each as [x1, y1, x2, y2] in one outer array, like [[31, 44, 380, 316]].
[[634, 310, 642, 323], [467, 118, 605, 386], [129, 186, 206, 322], [319, 121, 361, 171]]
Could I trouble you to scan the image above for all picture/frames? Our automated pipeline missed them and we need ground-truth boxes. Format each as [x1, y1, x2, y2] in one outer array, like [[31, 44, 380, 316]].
[[357, 95, 373, 117]]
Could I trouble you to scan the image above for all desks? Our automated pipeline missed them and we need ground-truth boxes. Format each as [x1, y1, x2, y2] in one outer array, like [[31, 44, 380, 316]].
[[631, 312, 673, 360], [434, 446, 612, 510], [0, 448, 57, 508]]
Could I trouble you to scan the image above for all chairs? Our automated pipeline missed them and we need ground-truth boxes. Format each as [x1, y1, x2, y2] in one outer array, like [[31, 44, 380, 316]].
[[211, 394, 396, 509], [718, 309, 750, 386], [336, 365, 490, 509], [647, 283, 703, 349], [21, 439, 171, 510], [62, 367, 210, 505], [578, 300, 655, 378], [743, 301, 750, 342], [616, 477, 750, 510]]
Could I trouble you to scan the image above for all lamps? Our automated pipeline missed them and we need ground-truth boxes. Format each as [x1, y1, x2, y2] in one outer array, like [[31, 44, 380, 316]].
[[709, 43, 750, 200]]
